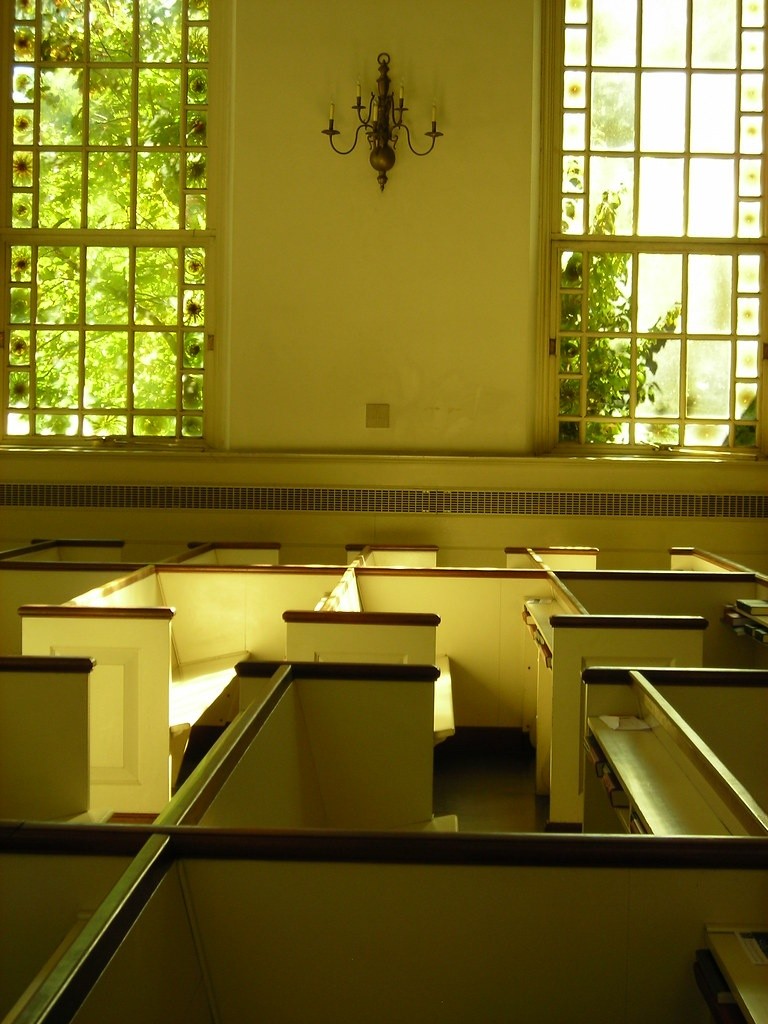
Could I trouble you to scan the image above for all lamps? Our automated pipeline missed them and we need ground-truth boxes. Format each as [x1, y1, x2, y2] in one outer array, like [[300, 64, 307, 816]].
[[321, 53, 444, 192]]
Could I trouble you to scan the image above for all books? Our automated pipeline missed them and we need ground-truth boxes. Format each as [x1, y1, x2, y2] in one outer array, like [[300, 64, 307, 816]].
[[723, 599, 768, 647], [522, 611, 552, 668], [583, 736, 648, 835], [692, 948, 749, 1024]]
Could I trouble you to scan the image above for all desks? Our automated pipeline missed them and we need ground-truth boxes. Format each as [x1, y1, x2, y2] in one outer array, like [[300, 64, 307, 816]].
[[0, 537, 768, 1024]]
